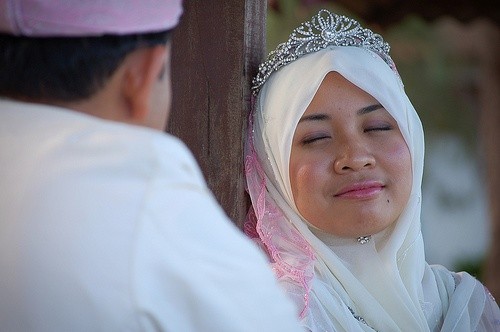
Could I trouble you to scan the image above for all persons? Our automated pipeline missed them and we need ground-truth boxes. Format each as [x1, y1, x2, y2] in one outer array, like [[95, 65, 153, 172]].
[[0, 0, 307, 332], [240, 10, 500, 332]]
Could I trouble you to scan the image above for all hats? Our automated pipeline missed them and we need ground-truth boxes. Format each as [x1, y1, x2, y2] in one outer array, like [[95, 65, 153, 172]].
[[0, 0, 184, 37]]
